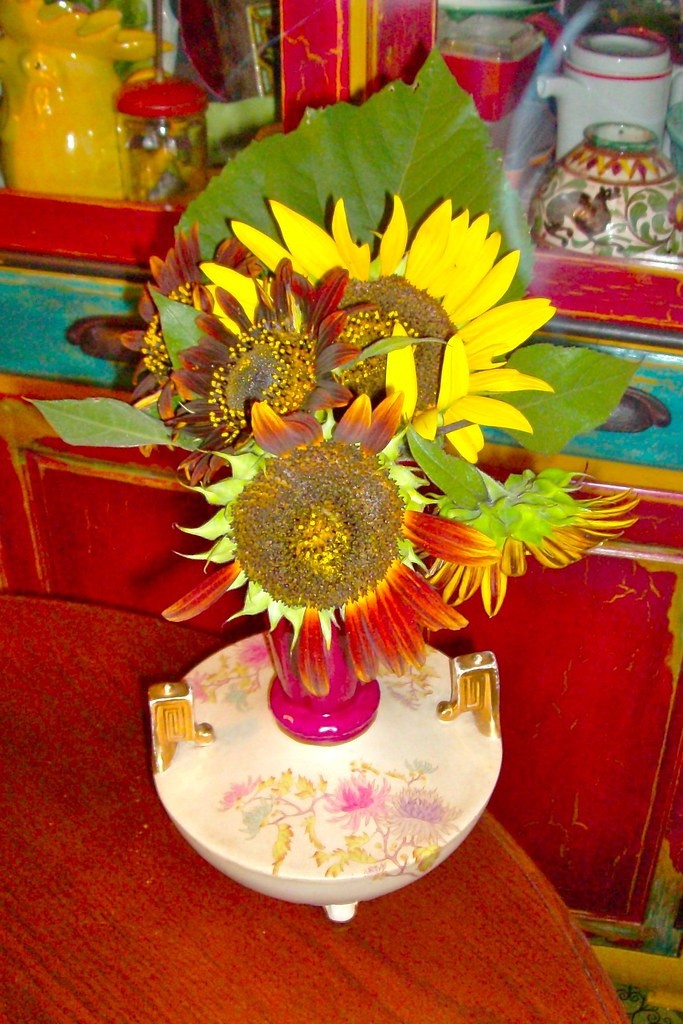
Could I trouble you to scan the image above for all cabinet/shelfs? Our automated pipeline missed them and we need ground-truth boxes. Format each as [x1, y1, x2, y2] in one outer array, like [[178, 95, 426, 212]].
[[0, 0, 683, 959]]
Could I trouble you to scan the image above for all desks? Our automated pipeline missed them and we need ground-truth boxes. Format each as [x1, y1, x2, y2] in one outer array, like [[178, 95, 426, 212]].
[[1, 596, 631, 1024]]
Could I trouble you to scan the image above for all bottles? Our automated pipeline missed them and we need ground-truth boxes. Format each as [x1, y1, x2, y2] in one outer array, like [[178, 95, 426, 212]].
[[527, 123, 683, 261], [117, 68, 208, 209]]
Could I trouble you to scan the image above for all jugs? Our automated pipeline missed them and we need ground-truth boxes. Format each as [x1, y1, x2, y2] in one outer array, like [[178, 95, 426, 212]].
[[538, 32, 683, 162]]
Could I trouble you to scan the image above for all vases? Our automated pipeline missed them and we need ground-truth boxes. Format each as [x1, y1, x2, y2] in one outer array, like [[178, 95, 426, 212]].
[[148, 598, 503, 923]]
